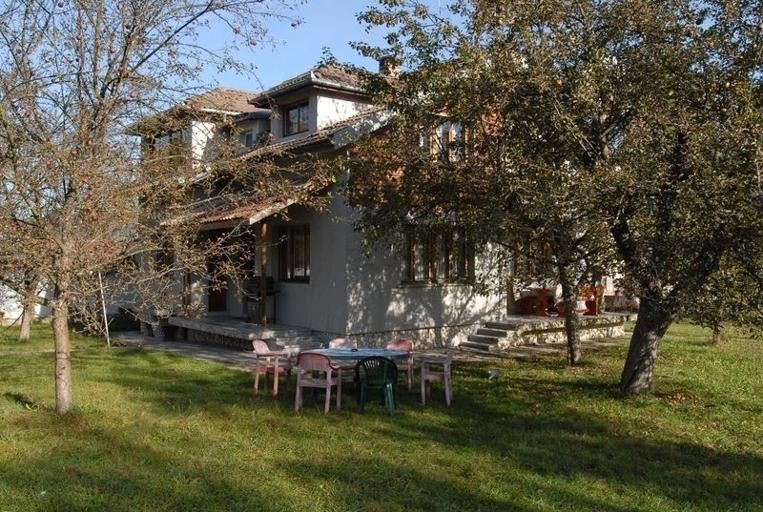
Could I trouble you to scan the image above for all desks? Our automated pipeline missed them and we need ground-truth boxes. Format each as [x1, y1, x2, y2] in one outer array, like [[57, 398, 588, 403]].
[[241, 276, 280, 324]]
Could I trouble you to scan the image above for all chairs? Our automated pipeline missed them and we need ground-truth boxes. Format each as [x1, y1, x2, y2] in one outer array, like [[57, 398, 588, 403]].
[[330, 338, 358, 390], [385, 337, 414, 391], [295, 352, 342, 414], [252, 339, 291, 400], [421, 349, 454, 407], [355, 356, 397, 416]]
